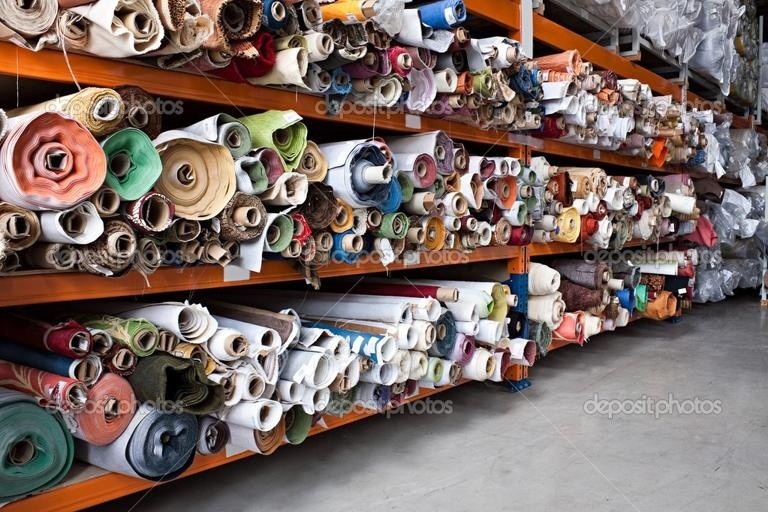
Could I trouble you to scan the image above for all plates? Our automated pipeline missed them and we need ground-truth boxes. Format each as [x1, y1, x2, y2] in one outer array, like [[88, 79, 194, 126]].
[[0, 0, 768, 512]]
